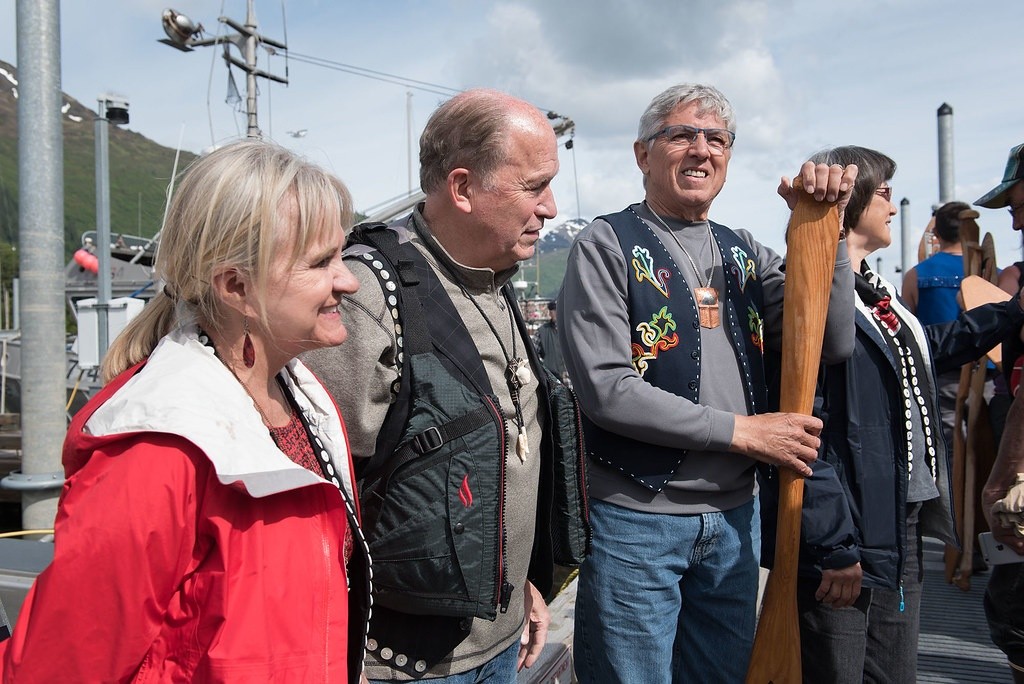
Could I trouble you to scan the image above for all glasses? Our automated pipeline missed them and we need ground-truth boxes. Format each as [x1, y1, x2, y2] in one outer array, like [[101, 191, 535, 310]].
[[874, 187, 892, 202], [648, 125, 735, 148]]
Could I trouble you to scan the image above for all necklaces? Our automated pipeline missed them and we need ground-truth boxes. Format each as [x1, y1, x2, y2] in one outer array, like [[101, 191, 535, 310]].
[[646, 201, 720, 329], [217, 351, 318, 476], [411, 213, 530, 462]]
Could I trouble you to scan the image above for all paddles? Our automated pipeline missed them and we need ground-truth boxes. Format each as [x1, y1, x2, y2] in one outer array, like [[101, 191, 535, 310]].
[[745, 176, 841, 684], [944, 241, 982, 586], [958, 233, 1000, 594]]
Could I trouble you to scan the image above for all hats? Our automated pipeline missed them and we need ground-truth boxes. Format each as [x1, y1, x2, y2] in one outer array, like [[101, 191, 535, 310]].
[[973, 143, 1024, 209]]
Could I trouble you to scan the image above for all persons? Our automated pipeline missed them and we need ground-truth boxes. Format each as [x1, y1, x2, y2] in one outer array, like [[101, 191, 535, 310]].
[[0, 139, 374, 684], [295, 88, 558, 684], [532, 83, 1024, 684]]
